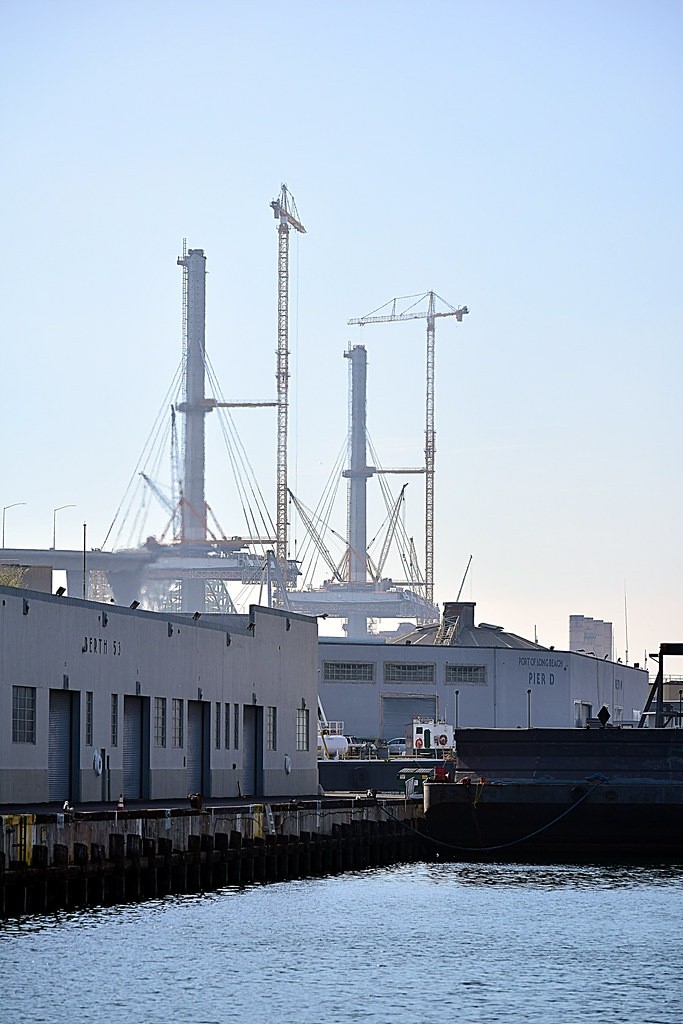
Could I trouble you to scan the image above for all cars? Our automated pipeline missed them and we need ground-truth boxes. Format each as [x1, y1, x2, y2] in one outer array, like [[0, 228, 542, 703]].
[[371, 735, 406, 755]]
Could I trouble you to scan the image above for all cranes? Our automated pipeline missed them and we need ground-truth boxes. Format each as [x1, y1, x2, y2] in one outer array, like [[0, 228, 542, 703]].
[[347, 288, 469, 624], [270, 181, 307, 611]]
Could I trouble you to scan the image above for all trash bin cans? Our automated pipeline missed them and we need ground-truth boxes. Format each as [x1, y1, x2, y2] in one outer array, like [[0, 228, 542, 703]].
[[397, 767, 435, 794]]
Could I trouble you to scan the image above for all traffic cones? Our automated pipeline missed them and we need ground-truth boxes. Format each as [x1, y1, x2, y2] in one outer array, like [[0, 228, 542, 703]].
[[114, 794, 124, 811]]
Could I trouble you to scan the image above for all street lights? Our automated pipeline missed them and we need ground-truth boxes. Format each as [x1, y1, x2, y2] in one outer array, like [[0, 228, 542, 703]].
[[53, 504, 77, 550], [2, 502, 27, 549], [83, 520, 87, 600]]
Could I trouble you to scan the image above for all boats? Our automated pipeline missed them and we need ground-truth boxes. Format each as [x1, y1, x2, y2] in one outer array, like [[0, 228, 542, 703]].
[[316, 696, 460, 791]]
